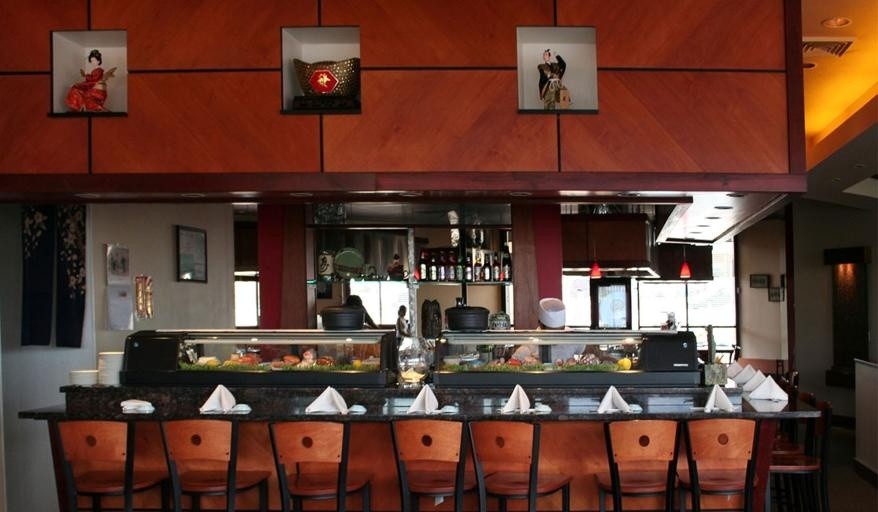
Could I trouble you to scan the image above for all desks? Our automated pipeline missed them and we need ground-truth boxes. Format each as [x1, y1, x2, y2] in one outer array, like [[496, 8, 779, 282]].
[[697, 343, 734, 364]]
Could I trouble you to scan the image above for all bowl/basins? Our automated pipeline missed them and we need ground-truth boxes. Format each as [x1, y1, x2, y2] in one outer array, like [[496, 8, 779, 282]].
[[445, 305, 489, 330], [320, 307, 365, 330]]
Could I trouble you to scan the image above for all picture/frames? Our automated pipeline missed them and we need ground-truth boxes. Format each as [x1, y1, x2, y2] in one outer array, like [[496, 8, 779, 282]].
[[174, 222, 211, 284], [749, 272, 787, 304]]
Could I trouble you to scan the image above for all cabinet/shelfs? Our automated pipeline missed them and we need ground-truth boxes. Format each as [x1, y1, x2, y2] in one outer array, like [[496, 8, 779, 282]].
[[303, 222, 414, 338], [432, 324, 702, 389], [412, 224, 513, 340], [121, 325, 401, 389]]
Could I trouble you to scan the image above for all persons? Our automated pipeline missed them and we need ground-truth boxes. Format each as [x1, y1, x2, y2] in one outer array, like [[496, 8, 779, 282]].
[[537, 48, 573, 108], [395, 305, 410, 342], [65, 49, 117, 111], [512, 298, 615, 364]]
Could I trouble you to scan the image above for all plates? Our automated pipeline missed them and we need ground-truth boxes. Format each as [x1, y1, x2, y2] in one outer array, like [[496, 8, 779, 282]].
[[66, 351, 125, 387], [120, 400, 732, 414]]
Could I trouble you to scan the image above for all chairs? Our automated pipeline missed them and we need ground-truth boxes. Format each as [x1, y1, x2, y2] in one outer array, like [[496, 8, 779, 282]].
[[762, 366, 835, 511], [49, 411, 171, 511], [157, 413, 273, 511], [464, 411, 574, 511], [266, 414, 375, 511], [386, 415, 498, 511], [672, 411, 762, 511], [733, 345, 742, 363], [592, 413, 684, 511]]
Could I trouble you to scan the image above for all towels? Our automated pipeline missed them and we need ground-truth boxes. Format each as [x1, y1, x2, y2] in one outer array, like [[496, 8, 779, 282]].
[[700, 382, 731, 412], [726, 357, 790, 402], [303, 386, 352, 417], [595, 384, 636, 414], [500, 381, 534, 413], [198, 382, 238, 413], [118, 393, 156, 414], [403, 383, 439, 414]]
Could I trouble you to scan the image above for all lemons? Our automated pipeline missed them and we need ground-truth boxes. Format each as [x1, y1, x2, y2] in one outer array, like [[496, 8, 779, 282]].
[[617, 358, 631, 371], [352, 360, 361, 369], [206, 360, 216, 367]]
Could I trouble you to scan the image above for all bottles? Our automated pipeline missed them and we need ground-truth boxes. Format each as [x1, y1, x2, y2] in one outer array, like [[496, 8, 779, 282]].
[[414, 246, 513, 283]]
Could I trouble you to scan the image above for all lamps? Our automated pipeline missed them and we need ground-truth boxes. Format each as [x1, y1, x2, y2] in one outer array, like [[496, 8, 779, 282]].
[[584, 204, 605, 280], [678, 244, 694, 279]]
